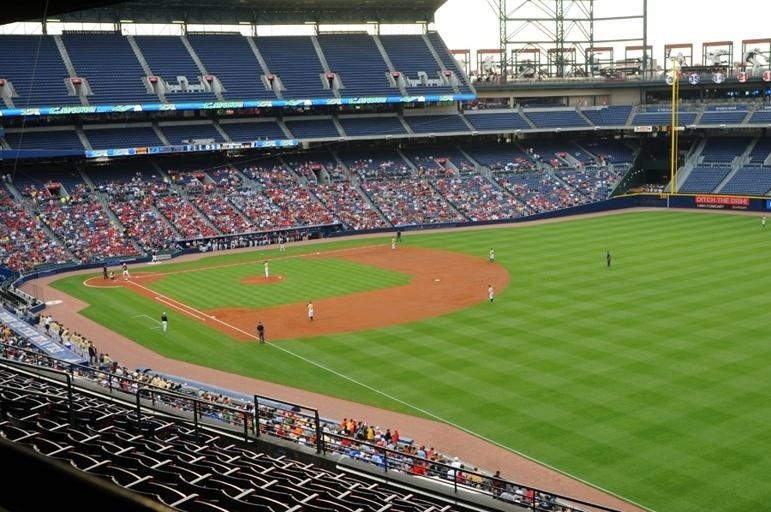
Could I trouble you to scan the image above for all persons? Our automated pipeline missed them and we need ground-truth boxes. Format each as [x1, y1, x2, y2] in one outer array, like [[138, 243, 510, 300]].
[[121, 262, 130, 280], [0, 144, 665, 274], [0, 299, 572, 512], [760, 213, 767, 227], [109, 271, 116, 280], [264, 260, 269, 277], [488, 285, 494, 303], [103, 265, 108, 280]]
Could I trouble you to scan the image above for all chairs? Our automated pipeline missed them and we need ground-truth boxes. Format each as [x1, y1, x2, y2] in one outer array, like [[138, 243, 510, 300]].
[[1, 371, 452, 512]]
